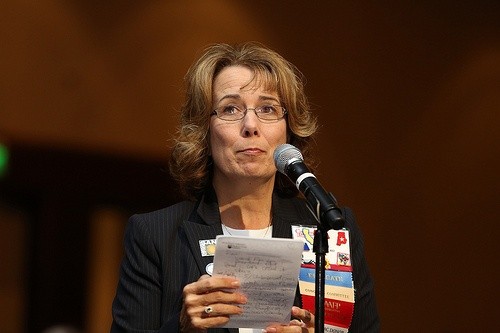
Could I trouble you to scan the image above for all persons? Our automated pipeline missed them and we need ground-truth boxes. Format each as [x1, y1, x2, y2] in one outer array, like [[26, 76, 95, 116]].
[[111, 40, 379, 333]]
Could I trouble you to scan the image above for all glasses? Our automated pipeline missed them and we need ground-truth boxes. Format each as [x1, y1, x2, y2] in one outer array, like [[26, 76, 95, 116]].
[[209, 105, 290, 121]]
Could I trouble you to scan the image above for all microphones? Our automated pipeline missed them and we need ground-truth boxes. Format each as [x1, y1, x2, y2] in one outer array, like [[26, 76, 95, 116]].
[[272, 143, 345, 231]]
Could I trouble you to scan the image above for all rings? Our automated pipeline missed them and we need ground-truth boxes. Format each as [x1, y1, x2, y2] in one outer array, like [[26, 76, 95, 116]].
[[205, 305, 213, 317]]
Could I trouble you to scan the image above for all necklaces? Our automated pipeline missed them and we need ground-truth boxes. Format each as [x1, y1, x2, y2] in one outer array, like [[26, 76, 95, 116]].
[[222, 212, 272, 238]]
